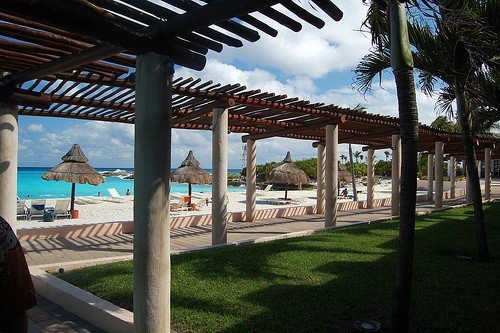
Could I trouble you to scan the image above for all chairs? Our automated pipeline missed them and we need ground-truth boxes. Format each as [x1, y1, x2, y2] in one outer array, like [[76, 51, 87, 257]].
[[17, 200, 72, 220]]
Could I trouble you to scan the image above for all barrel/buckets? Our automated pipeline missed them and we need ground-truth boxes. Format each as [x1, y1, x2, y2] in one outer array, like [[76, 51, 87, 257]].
[[190, 204, 196, 211], [71, 210, 78, 219], [45, 212, 52, 222], [184, 196, 191, 202]]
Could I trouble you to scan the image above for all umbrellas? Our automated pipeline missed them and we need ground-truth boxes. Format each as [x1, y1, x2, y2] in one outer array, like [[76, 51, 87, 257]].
[[338, 161, 353, 196], [269, 151, 307, 200], [171, 150, 213, 207], [41, 143, 106, 210]]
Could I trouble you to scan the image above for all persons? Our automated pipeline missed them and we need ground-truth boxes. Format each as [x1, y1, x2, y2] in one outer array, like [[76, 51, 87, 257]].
[[97, 192, 101, 196], [126, 189, 130, 196], [0, 216, 38, 333], [341, 188, 348, 198]]
